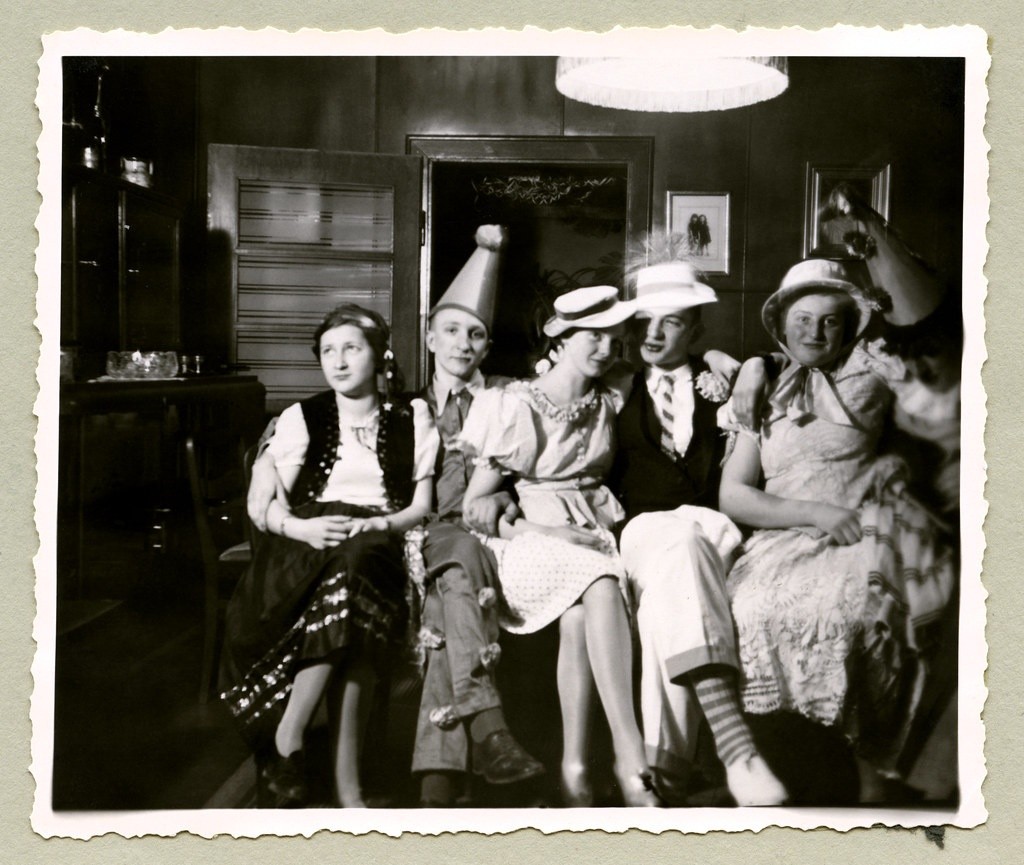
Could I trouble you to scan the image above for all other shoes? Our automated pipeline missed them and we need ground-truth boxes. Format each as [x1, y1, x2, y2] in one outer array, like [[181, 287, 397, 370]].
[[724, 751, 789, 806], [614, 760, 663, 807], [562, 782, 593, 807], [254, 732, 309, 800]]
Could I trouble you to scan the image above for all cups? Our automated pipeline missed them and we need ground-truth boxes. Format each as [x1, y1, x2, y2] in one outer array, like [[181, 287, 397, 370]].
[[105, 350, 202, 378]]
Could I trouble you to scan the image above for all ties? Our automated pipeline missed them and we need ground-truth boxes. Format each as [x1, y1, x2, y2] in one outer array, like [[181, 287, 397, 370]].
[[659, 375, 678, 466]]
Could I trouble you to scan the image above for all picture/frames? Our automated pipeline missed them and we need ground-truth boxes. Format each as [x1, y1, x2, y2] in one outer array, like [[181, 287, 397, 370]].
[[666, 190, 732, 276], [801, 160, 891, 263]]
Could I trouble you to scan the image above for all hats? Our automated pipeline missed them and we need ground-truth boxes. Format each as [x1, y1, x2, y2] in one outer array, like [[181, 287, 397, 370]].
[[629, 262, 720, 319], [427, 223, 506, 338], [761, 258, 872, 344], [825, 183, 943, 326], [542, 285, 641, 337]]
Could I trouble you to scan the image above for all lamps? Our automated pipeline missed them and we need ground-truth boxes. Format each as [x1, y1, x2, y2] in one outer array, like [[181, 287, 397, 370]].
[[555, 56, 790, 112]]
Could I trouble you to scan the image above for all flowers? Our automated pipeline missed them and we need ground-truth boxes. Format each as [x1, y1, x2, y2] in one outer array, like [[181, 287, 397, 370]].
[[696, 370, 729, 403]]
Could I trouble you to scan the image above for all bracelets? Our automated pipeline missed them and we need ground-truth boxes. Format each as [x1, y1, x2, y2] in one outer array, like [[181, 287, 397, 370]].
[[382, 515, 392, 530], [281, 514, 295, 538], [749, 351, 777, 376]]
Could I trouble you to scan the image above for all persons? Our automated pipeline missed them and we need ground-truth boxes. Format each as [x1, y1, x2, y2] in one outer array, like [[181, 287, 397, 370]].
[[412, 224, 547, 808], [687, 214, 712, 258], [717, 259, 961, 807], [459, 285, 741, 807], [602, 230, 788, 808], [246, 301, 441, 808]]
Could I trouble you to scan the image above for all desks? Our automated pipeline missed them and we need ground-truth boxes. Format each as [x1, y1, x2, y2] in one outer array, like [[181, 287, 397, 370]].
[[66, 375, 266, 614]]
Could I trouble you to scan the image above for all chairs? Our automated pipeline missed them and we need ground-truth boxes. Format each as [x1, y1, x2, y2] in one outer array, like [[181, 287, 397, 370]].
[[186, 431, 254, 705], [243, 443, 391, 808]]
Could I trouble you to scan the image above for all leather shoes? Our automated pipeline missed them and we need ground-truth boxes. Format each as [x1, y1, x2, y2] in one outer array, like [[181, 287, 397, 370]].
[[417, 789, 457, 808], [471, 730, 545, 785]]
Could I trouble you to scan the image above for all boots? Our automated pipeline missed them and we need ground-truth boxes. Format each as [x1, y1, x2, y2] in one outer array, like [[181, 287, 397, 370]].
[[845, 702, 887, 802]]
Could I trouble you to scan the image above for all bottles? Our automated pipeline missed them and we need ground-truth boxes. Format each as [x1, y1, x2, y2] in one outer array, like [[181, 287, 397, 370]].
[[82, 104, 107, 171]]
